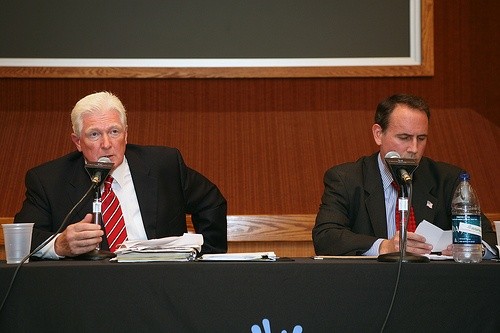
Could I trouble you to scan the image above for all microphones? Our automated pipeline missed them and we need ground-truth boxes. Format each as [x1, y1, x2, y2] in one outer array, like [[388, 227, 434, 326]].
[[385, 151, 413, 183], [91, 157, 112, 185]]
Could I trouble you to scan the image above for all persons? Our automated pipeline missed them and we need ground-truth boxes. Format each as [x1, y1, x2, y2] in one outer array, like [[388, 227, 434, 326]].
[[312, 94, 500, 260], [14, 92, 228, 261]]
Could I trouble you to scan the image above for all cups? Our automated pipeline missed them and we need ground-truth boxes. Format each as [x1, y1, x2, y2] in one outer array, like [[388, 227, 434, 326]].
[[493, 220, 500, 247], [0, 223, 35, 264]]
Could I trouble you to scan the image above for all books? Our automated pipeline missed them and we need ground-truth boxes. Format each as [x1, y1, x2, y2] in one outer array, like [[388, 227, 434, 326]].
[[117, 248, 197, 262]]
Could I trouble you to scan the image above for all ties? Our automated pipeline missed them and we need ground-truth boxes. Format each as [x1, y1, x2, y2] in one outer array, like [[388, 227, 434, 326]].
[[391, 180, 416, 232], [101, 175, 129, 253]]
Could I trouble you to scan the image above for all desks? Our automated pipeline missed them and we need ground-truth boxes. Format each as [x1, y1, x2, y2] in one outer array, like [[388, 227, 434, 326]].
[[0, 254, 500, 333]]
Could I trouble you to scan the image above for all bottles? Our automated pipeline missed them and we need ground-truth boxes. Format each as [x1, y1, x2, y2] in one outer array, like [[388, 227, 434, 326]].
[[450, 174, 483, 264]]
